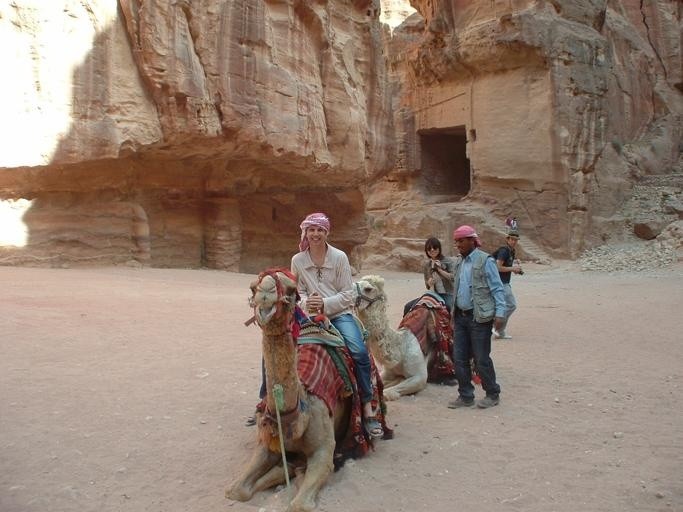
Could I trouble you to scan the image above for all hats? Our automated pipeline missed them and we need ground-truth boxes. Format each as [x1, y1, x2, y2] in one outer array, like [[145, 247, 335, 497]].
[[506, 230, 520, 240]]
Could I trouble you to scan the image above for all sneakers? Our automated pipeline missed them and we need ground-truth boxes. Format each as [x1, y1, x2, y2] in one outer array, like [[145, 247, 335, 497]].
[[478, 398, 500, 408], [448, 395, 475, 408], [492, 327, 512, 339]]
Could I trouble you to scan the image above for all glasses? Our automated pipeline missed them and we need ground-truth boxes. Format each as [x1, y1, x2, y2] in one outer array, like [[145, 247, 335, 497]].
[[427, 245, 438, 251]]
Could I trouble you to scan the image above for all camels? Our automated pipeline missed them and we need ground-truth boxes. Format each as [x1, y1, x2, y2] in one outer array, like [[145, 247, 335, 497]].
[[223, 270, 381, 512], [351, 274, 476, 402]]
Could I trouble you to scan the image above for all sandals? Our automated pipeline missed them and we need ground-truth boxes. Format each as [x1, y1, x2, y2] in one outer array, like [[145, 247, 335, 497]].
[[245, 405, 264, 426], [364, 416, 385, 439]]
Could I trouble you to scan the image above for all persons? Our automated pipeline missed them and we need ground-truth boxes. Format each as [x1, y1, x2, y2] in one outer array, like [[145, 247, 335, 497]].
[[492, 231, 523, 339], [404, 238, 455, 317], [246, 212, 386, 439], [447, 226, 506, 408], [511, 217, 517, 229]]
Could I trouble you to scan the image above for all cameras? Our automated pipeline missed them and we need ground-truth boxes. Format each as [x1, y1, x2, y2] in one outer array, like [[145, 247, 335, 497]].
[[520, 268, 524, 275]]
